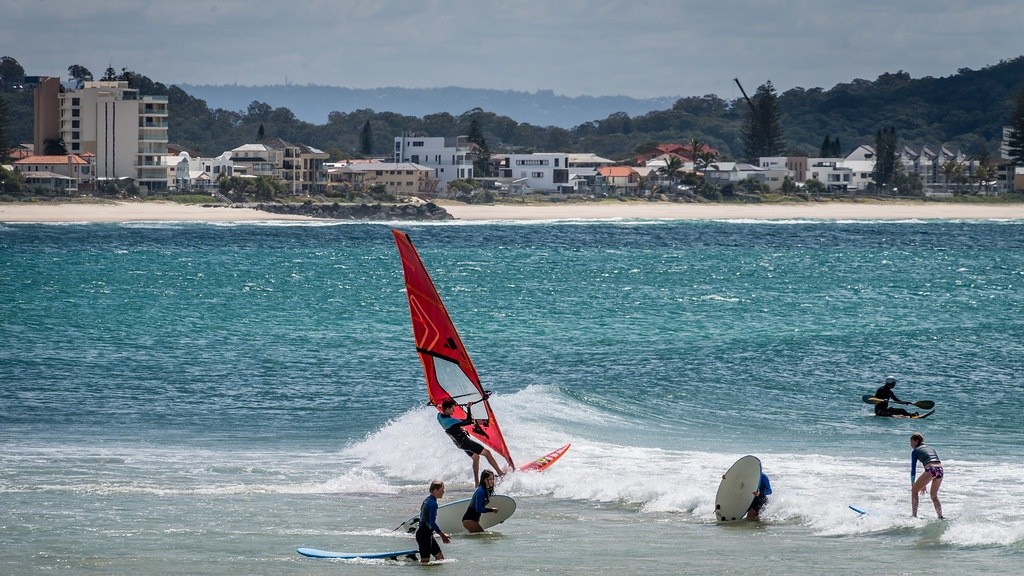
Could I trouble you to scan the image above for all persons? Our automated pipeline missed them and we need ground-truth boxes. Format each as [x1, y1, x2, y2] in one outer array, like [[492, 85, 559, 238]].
[[875, 376, 919, 417], [722, 471, 772, 520], [910, 433, 944, 518], [416, 481, 451, 562], [437, 399, 506, 488], [462, 469, 498, 533]]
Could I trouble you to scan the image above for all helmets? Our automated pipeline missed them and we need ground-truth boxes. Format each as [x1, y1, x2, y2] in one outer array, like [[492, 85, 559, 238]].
[[886, 376, 897, 383]]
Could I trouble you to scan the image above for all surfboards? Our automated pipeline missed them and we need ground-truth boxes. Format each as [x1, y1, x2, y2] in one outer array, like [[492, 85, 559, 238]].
[[713, 456, 763, 524], [295, 546, 420, 561], [893, 410, 935, 420], [848, 504, 867, 515], [404, 494, 515, 535]]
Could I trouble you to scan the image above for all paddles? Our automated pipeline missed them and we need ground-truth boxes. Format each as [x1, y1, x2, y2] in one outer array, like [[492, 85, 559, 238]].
[[860, 393, 936, 412]]
